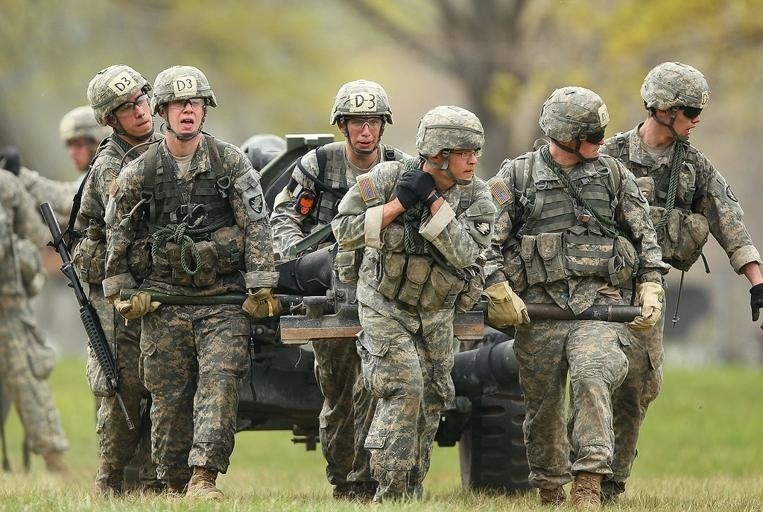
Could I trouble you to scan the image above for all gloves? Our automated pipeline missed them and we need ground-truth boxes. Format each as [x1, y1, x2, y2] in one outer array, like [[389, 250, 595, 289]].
[[629, 281, 665, 328], [110, 290, 161, 319], [397, 169, 435, 209], [750, 283, 763, 329], [242, 289, 283, 319], [483, 280, 531, 328]]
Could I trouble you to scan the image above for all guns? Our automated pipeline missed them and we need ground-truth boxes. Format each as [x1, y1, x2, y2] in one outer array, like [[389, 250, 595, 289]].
[[41, 203, 134, 431]]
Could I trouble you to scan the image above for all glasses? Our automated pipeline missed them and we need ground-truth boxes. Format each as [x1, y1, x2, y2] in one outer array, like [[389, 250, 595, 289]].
[[678, 106, 703, 118], [579, 131, 603, 145], [349, 117, 385, 129], [448, 149, 481, 159]]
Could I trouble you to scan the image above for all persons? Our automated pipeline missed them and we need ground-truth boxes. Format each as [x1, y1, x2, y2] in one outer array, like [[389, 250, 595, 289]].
[[75, 65, 165, 498], [0, 169, 70, 472], [484, 86, 670, 512], [0, 106, 113, 228], [331, 105, 497, 503], [598, 62, 763, 505], [104, 66, 279, 500], [268, 80, 416, 502]]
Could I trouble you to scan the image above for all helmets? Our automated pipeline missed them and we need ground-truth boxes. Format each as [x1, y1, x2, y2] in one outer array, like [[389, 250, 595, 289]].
[[60, 64, 217, 145], [539, 87, 610, 143], [330, 80, 394, 125], [416, 107, 485, 158], [641, 62, 709, 111]]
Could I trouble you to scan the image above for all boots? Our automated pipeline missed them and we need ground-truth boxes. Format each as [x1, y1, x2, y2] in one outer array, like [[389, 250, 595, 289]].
[[96, 466, 123, 499], [186, 466, 224, 500], [540, 485, 566, 505], [570, 471, 603, 512]]
